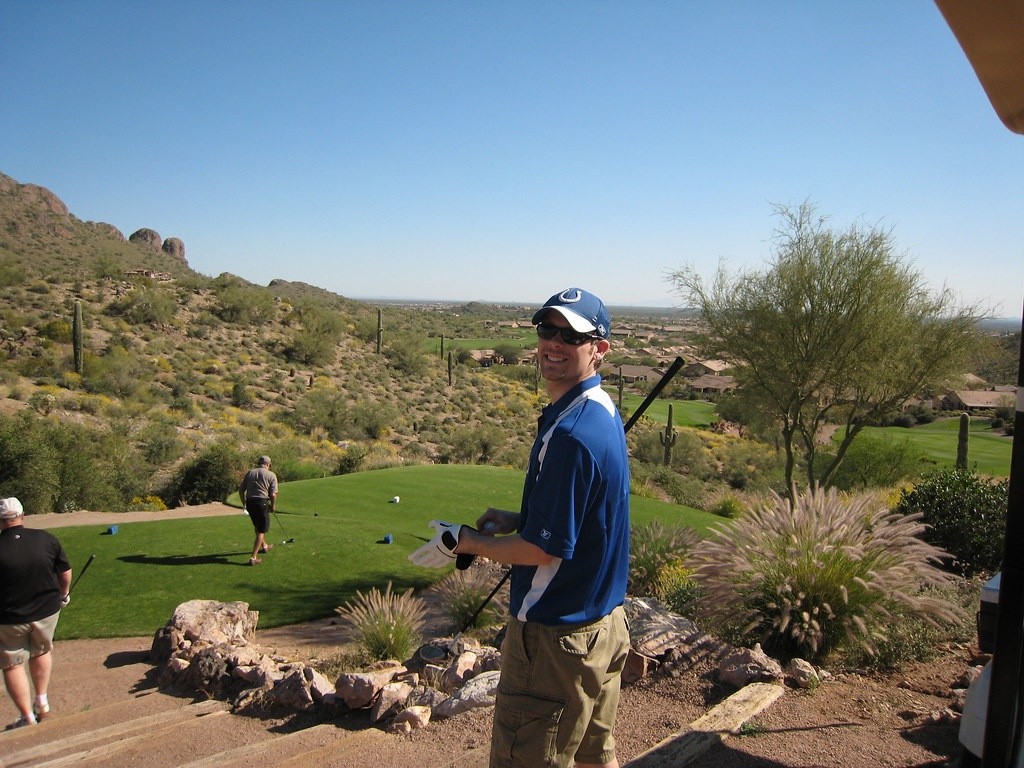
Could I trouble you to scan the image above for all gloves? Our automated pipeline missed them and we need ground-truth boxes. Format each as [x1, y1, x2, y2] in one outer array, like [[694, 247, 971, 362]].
[[407, 519, 478, 570], [60, 592, 70, 608]]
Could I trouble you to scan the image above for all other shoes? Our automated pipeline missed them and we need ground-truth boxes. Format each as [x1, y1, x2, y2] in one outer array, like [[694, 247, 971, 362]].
[[249, 558, 262, 566], [32, 710, 49, 723], [263, 544, 273, 553], [7, 718, 38, 730]]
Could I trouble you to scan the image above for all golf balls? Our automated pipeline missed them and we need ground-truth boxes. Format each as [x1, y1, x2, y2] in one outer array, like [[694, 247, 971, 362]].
[[282, 541, 286, 544]]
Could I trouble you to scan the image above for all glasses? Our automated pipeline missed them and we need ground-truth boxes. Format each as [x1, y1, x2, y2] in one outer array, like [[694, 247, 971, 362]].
[[537, 324, 605, 345]]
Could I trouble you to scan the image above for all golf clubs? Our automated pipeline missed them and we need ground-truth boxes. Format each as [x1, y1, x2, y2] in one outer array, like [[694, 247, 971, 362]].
[[268, 504, 296, 542], [58, 554, 96, 602], [418, 356, 686, 663]]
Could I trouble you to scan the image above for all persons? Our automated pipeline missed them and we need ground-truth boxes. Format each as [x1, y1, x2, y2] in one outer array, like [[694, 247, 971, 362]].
[[239, 455, 278, 564], [408, 288, 630, 768], [0, 497, 72, 729]]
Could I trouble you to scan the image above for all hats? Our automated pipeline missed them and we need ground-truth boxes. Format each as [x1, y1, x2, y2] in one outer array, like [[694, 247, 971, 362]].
[[0, 497, 23, 519], [532, 288, 611, 339], [258, 456, 272, 468]]
